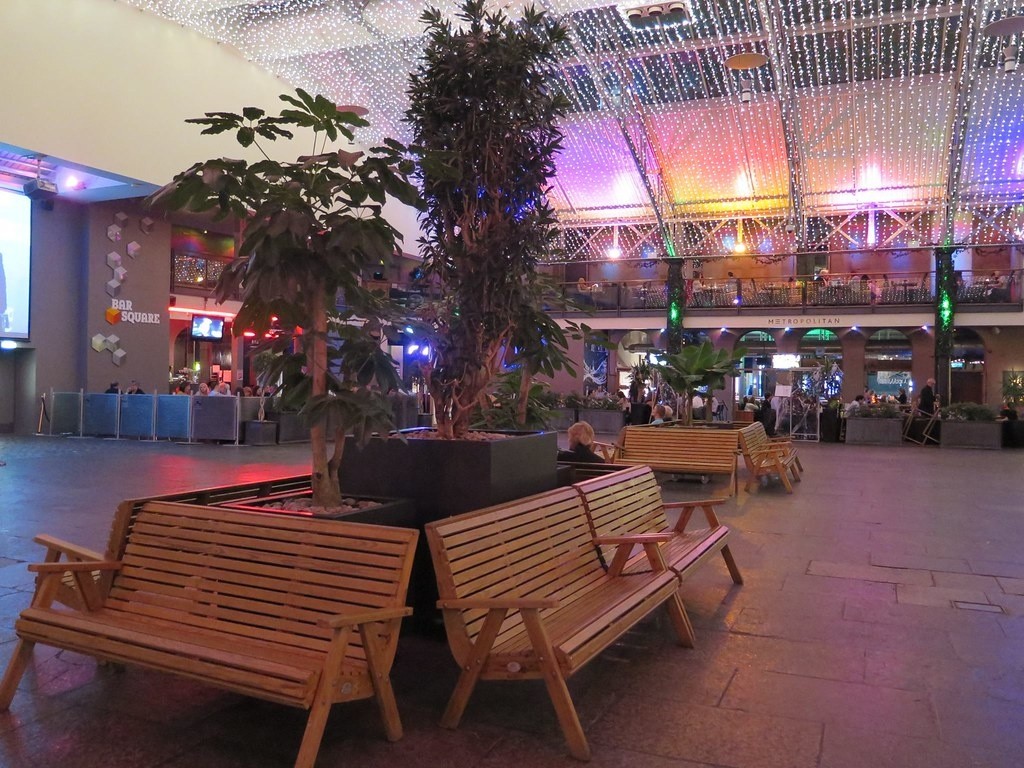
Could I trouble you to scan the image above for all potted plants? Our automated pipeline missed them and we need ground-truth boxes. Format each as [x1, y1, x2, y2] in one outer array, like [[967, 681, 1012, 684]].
[[340, 0, 568, 637], [577, 399, 626, 433], [939, 401, 1006, 449], [540, 390, 577, 429], [244, 351, 278, 446], [844, 402, 905, 446], [142, 85, 428, 675]]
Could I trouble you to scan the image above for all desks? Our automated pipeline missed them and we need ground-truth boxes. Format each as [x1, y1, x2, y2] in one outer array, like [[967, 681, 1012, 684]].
[[765, 286, 786, 304], [974, 281, 998, 303], [705, 288, 725, 306], [894, 282, 918, 302]]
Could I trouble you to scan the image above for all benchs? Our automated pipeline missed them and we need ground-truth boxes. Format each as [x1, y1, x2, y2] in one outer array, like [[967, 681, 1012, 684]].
[[423, 487, 694, 762], [738, 427, 801, 495], [735, 403, 755, 422], [592, 420, 674, 463], [574, 465, 742, 643], [32, 472, 314, 670], [750, 421, 804, 482], [667, 418, 682, 427], [0, 499, 419, 768], [557, 462, 636, 482], [612, 427, 739, 498], [693, 419, 753, 453]]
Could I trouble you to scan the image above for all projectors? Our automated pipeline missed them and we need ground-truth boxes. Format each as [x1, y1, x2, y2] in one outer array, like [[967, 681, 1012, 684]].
[[23, 178, 58, 200]]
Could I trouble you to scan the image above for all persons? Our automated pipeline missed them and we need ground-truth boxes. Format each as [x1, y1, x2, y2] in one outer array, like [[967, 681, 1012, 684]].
[[955, 270, 1007, 303], [692, 271, 794, 305], [577, 277, 668, 310], [651, 381, 719, 424], [169, 364, 281, 397], [995, 402, 1018, 422], [557, 421, 605, 463], [630, 371, 644, 404], [814, 270, 896, 303], [556, 388, 631, 413], [846, 387, 907, 417], [127, 379, 145, 394], [919, 378, 938, 416], [104, 381, 124, 394], [744, 392, 780, 437]]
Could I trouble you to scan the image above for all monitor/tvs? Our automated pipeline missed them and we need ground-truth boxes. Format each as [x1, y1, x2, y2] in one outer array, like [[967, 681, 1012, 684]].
[[190, 313, 225, 342]]
